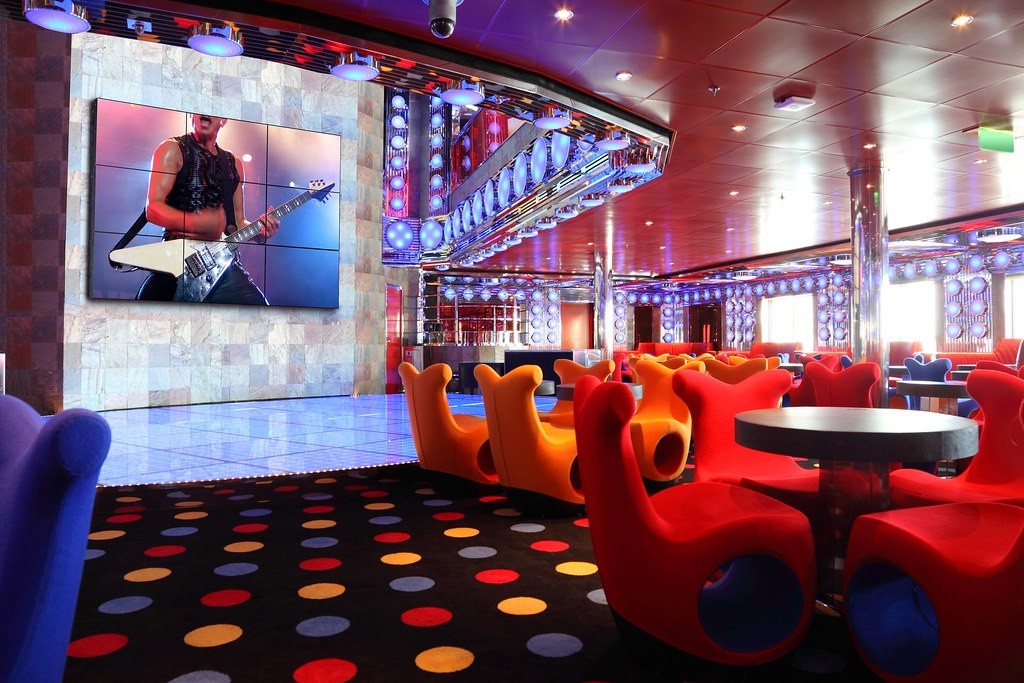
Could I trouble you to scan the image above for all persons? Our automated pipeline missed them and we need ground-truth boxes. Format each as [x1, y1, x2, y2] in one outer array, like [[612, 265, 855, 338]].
[[136, 113, 280, 307]]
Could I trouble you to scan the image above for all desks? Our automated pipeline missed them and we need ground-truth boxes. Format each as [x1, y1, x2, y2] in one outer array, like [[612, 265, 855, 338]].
[[554, 382, 642, 402], [956, 363, 1017, 372], [887, 364, 915, 375], [734, 407, 978, 618], [896, 380, 975, 420], [951, 371, 971, 383], [778, 362, 804, 372]]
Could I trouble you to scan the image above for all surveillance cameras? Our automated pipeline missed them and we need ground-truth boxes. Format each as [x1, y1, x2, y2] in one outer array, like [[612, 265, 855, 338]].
[[429, 19, 455, 38]]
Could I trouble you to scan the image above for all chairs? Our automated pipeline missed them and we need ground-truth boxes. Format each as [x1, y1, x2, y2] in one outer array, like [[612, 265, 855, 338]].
[[395, 339, 1024, 683], [0, 392, 111, 683]]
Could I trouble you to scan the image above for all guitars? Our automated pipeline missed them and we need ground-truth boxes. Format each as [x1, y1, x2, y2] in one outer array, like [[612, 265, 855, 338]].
[[107, 178, 336, 302]]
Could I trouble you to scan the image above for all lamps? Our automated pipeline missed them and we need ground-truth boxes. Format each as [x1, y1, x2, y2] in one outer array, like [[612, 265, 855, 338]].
[[188, 22, 245, 58], [378, 80, 1011, 340], [328, 51, 380, 82], [21, 0, 91, 35]]
